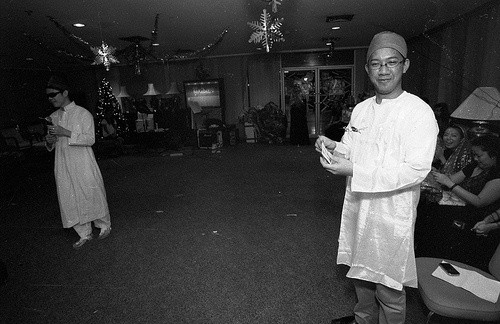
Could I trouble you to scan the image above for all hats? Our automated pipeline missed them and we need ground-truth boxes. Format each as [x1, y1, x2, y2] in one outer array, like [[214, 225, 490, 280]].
[[366, 30, 408, 63]]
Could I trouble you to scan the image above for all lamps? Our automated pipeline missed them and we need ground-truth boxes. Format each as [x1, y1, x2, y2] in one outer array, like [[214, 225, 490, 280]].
[[117, 85, 130, 97], [166, 82, 181, 95], [449, 87, 500, 137], [143, 83, 161, 96]]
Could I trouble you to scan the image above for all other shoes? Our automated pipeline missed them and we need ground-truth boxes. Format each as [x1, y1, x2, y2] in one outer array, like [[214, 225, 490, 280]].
[[77, 235, 92, 248], [98, 229, 110, 240]]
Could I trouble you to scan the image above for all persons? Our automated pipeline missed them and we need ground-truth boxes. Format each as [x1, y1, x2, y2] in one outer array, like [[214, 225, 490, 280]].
[[289, 86, 311, 146], [315, 32, 439, 324], [44, 73, 112, 248], [414, 124, 500, 273], [325, 107, 352, 142]]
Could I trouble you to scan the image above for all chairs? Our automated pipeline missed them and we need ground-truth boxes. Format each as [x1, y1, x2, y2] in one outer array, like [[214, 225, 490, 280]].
[[415, 241, 500, 324], [0, 126, 52, 164]]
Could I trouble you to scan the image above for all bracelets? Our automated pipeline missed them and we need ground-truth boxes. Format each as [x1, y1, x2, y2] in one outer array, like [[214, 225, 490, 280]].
[[449, 183, 457, 190]]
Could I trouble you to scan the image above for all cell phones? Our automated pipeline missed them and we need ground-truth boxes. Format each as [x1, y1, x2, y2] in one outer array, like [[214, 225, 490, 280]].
[[438, 262, 460, 277]]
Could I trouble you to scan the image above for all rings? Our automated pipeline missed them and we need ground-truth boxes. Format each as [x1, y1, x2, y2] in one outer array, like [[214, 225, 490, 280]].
[[53, 128, 54, 129]]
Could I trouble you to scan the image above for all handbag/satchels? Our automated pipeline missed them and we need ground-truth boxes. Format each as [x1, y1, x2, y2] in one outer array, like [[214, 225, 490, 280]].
[[420, 175, 444, 204]]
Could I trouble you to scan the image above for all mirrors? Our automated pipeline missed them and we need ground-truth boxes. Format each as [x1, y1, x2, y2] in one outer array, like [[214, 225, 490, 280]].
[[183, 78, 224, 131]]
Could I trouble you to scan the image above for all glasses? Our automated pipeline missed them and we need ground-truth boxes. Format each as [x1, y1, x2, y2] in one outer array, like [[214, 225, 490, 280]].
[[46, 92, 60, 98], [368, 57, 409, 70]]
